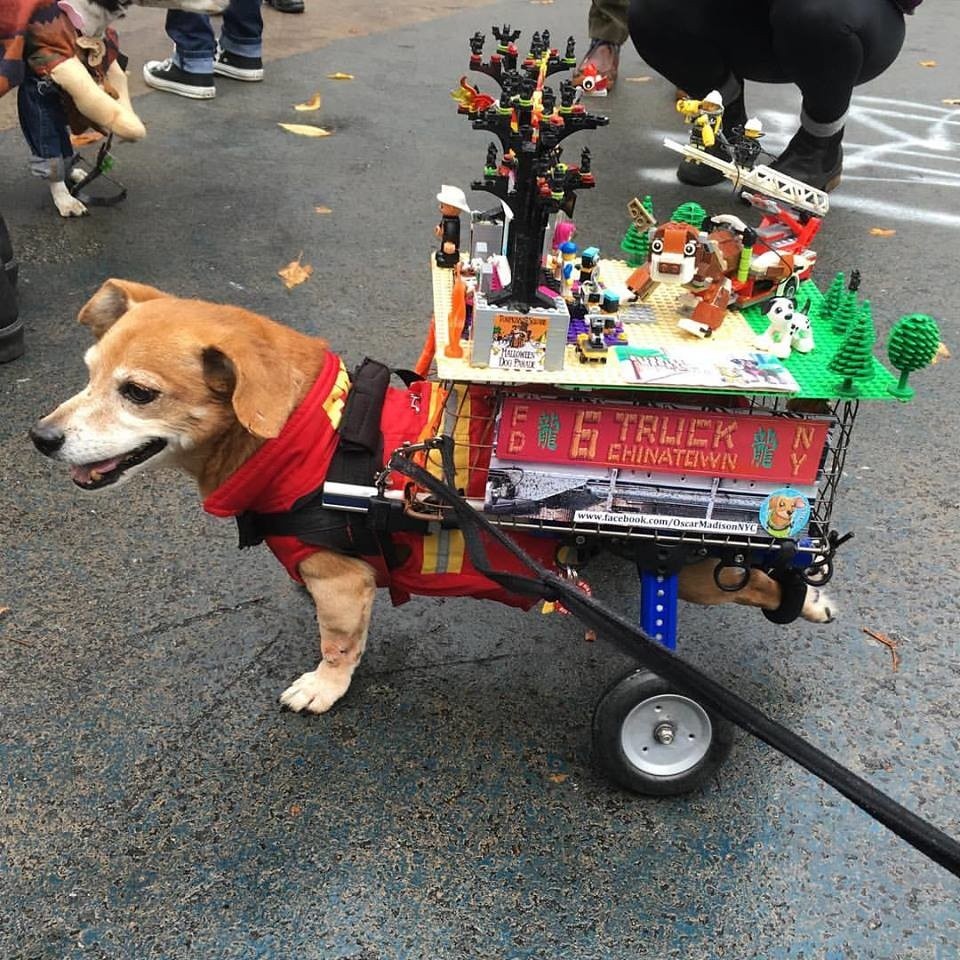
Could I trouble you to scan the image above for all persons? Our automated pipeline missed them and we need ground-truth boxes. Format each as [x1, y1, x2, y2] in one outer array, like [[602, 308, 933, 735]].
[[141, 0, 312, 101], [573, 1, 922, 207]]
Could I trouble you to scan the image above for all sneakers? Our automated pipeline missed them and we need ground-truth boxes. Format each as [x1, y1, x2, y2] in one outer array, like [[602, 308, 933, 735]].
[[143, 58, 216, 98], [211, 36, 265, 81]]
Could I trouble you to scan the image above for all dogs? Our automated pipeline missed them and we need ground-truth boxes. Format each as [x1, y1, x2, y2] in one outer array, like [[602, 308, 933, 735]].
[[753, 296, 815, 358], [29, 278, 837, 718], [17, 0, 147, 212]]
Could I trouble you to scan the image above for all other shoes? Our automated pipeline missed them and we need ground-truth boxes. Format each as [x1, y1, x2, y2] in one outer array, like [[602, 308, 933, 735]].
[[270, 0, 305, 12], [676, 96, 762, 188], [743, 125, 845, 204]]
